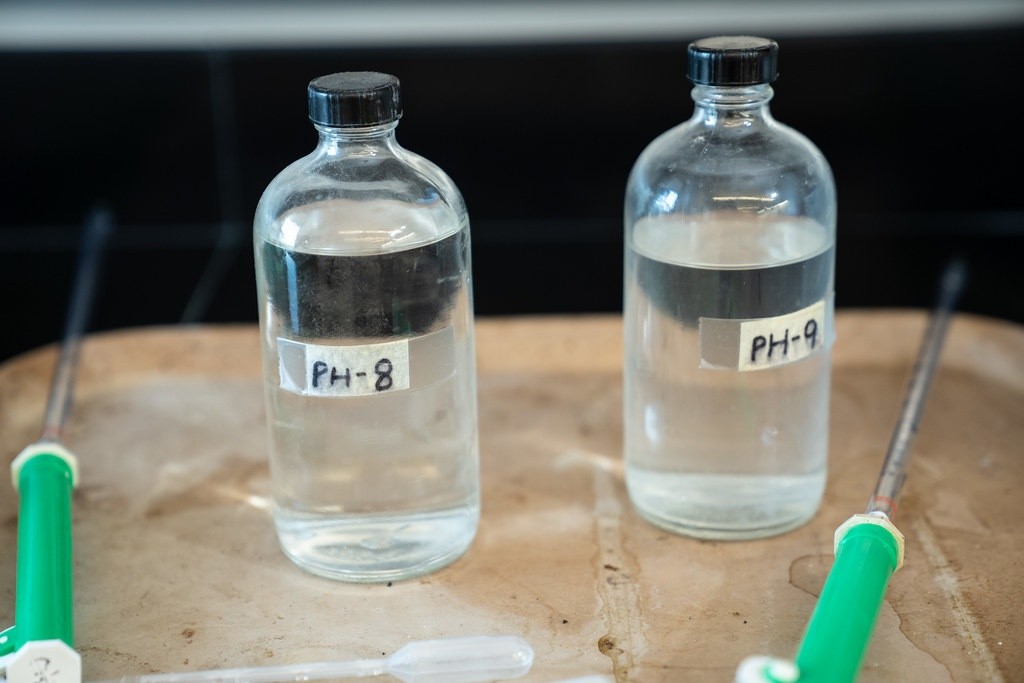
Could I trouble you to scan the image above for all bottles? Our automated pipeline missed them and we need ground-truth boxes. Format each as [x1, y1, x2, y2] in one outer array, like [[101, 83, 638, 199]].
[[251, 71, 484, 583], [618, 32, 838, 542]]
